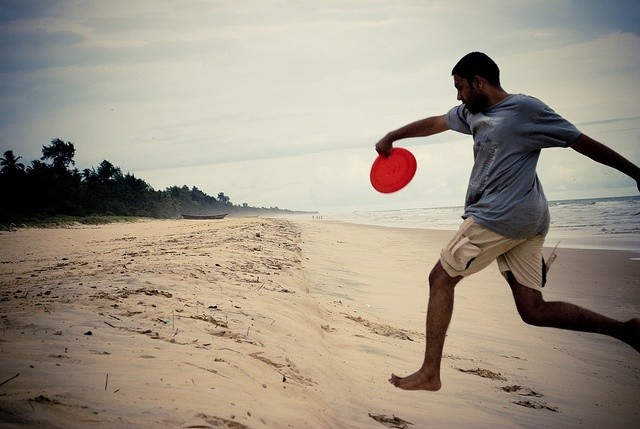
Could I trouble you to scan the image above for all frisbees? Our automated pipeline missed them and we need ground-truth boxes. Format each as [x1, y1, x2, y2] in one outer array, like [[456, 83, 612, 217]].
[[370, 148, 416, 193]]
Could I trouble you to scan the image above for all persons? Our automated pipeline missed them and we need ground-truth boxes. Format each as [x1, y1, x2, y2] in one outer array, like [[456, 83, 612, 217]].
[[375, 48, 640, 392]]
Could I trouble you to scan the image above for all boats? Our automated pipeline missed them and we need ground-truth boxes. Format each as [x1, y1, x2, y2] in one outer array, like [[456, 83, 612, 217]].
[[180, 213, 229, 219]]
